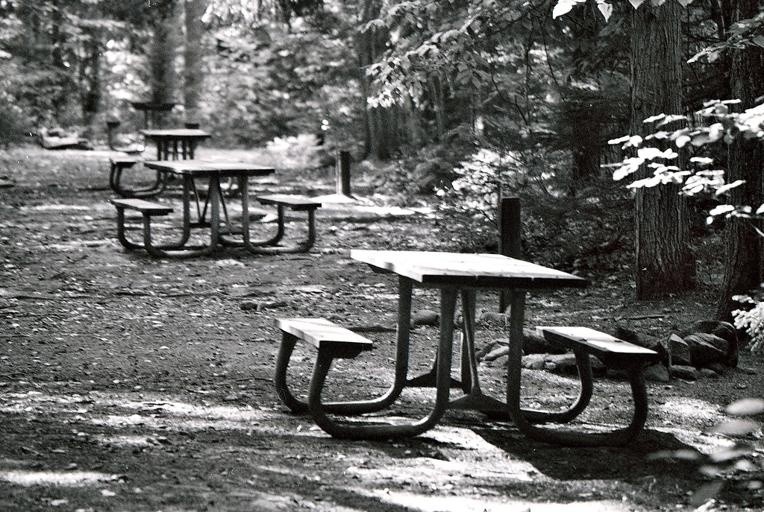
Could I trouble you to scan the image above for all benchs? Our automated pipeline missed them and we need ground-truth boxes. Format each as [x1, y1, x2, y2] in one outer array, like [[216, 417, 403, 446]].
[[256, 194, 324, 252], [110, 197, 175, 257], [535, 322, 659, 446], [272, 313, 376, 441], [108, 121, 243, 199]]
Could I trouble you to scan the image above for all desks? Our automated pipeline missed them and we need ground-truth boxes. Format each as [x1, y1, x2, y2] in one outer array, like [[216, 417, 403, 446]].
[[143, 157, 276, 258], [131, 102, 173, 149], [351, 246, 588, 446]]
